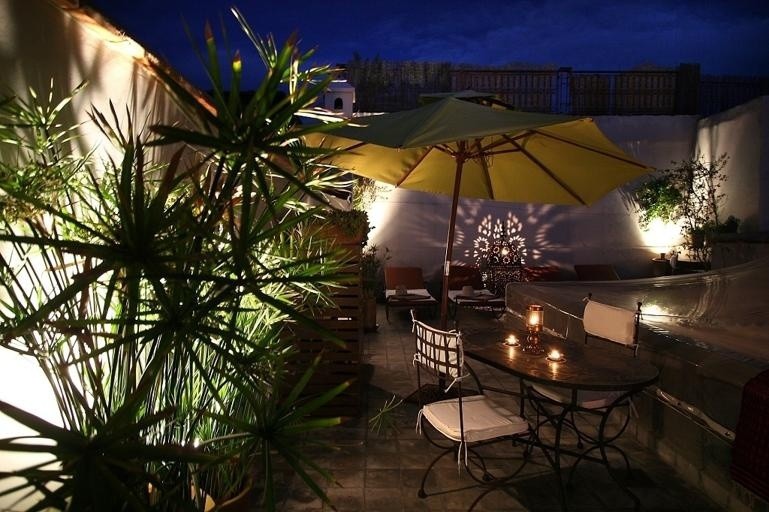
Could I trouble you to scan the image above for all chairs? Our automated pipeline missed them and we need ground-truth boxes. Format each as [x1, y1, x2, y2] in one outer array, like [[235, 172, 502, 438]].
[[441, 265, 506, 320], [529, 293, 643, 493], [526, 267, 564, 282], [410, 309, 537, 512], [574, 264, 620, 281], [386, 267, 439, 323]]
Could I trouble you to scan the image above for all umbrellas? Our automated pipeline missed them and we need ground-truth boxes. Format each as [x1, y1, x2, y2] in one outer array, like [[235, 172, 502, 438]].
[[287, 89, 656, 394]]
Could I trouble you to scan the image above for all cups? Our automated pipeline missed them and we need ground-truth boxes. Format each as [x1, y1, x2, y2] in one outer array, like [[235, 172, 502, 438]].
[[660, 252, 665, 260], [462, 285, 474, 296]]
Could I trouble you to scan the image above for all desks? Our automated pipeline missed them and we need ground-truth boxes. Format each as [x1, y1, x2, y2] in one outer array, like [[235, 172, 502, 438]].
[[667, 256, 709, 274], [460, 328, 660, 512]]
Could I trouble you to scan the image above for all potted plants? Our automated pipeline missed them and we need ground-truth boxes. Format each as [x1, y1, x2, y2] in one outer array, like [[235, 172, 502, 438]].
[[362, 245, 393, 329], [629, 148, 740, 258]]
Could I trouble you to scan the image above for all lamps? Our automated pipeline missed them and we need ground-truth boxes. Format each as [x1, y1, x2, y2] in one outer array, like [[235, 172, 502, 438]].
[[522, 304, 544, 356], [486, 221, 521, 266]]
